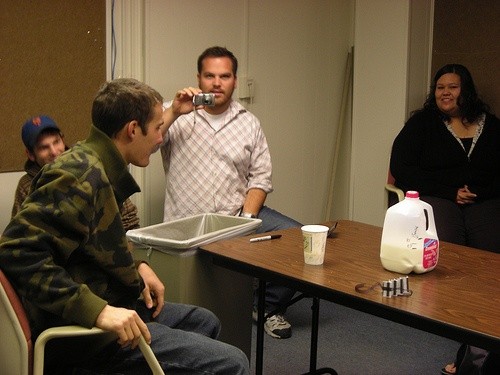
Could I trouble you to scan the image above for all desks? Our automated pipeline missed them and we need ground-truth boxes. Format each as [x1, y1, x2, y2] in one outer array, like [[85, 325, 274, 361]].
[[198, 219, 500, 375]]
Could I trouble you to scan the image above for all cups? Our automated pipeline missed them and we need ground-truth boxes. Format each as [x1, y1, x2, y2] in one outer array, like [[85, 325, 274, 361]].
[[300, 225, 330, 266]]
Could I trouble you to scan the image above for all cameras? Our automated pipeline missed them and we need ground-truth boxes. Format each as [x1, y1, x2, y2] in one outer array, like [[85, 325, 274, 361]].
[[195, 93, 215, 107]]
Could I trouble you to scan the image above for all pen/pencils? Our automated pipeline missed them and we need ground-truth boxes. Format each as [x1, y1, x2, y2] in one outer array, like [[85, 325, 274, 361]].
[[250, 234, 282, 243]]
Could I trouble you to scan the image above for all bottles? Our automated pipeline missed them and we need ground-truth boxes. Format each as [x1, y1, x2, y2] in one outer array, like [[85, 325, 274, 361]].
[[380, 191, 439, 274]]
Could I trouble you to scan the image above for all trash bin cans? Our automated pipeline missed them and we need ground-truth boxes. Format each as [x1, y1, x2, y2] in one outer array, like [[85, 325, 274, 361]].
[[126, 213, 263, 370]]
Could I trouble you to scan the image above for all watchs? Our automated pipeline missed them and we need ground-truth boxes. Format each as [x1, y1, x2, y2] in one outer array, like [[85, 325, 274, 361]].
[[240, 212, 255, 219]]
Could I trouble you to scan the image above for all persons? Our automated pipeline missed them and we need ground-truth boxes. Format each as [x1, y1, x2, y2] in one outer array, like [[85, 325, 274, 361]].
[[0, 77, 249, 375], [159, 45, 305, 338], [10, 114, 141, 243], [388, 64, 500, 254]]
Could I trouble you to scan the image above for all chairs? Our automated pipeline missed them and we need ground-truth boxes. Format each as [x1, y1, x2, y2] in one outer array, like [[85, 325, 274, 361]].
[[0, 269, 166, 375]]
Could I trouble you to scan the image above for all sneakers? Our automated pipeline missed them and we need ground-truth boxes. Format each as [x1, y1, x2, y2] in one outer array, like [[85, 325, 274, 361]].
[[252, 309, 291, 338]]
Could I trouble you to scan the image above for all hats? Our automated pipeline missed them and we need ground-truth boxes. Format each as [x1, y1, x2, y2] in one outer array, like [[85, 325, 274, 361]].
[[21, 116, 60, 152]]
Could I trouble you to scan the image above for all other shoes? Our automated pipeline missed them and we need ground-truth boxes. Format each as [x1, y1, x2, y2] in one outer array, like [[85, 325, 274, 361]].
[[440, 362, 457, 375]]
